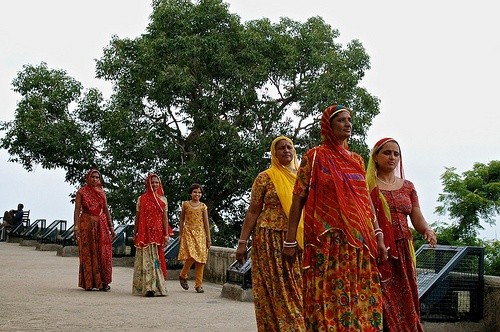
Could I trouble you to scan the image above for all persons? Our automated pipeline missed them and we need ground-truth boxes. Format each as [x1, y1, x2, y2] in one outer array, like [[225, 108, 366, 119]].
[[0, 203, 24, 242], [74, 170, 115, 291], [235, 105, 437, 332], [177, 184, 211, 293], [131, 174, 174, 297]]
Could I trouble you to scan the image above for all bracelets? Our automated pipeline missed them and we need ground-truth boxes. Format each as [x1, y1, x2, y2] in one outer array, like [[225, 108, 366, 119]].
[[374, 228, 383, 237], [283, 241, 298, 248], [74, 224, 79, 231], [424, 229, 435, 235], [238, 240, 247, 244]]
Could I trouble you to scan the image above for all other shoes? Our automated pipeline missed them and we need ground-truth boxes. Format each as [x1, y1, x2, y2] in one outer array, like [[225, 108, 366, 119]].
[[195, 287, 204, 293], [145, 291, 154, 298], [178, 274, 189, 290], [99, 285, 111, 291]]
